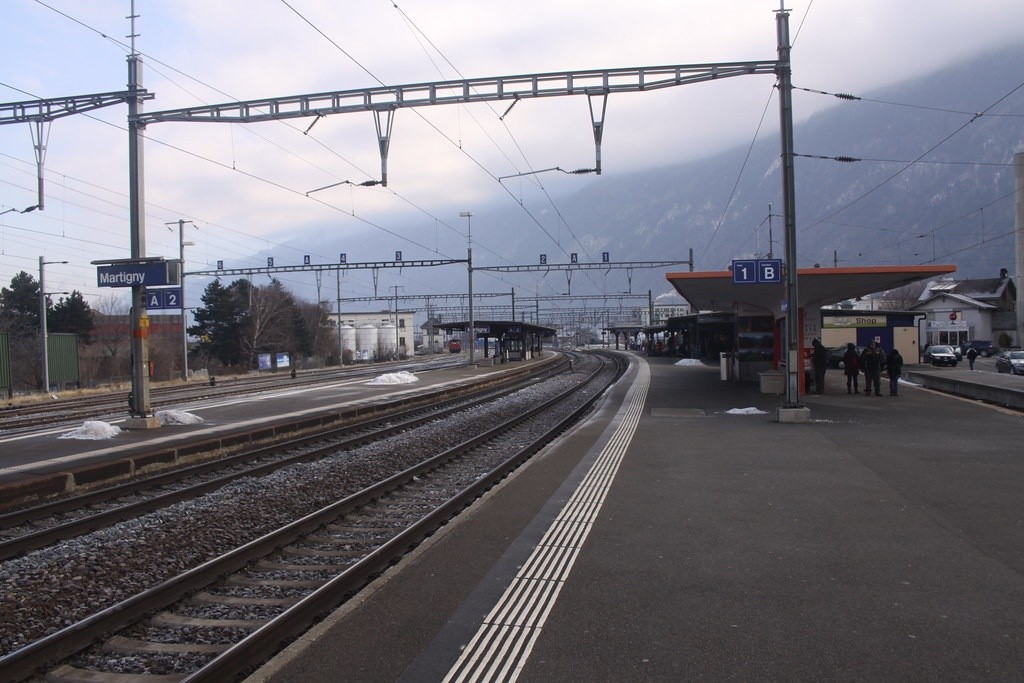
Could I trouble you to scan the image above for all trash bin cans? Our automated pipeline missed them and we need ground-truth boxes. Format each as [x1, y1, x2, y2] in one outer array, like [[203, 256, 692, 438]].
[[503, 350, 510, 362], [720, 351, 733, 380]]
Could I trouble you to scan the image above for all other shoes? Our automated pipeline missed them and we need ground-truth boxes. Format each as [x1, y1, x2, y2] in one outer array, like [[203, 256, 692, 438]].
[[875, 393, 882, 396], [890, 392, 899, 396], [864, 392, 870, 396], [848, 391, 852, 394], [854, 390, 860, 394]]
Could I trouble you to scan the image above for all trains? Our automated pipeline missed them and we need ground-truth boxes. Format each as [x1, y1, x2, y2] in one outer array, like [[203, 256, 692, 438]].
[[449, 339, 461, 353]]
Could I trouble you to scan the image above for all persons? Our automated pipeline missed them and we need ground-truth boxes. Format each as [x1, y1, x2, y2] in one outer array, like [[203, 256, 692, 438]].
[[886, 349, 903, 396], [967, 345, 978, 370], [810, 338, 827, 395], [860, 340, 886, 396], [844, 343, 861, 394]]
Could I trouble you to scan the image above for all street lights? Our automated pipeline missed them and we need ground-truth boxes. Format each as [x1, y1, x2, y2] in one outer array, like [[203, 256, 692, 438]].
[[39, 256, 69, 393], [179, 242, 196, 377]]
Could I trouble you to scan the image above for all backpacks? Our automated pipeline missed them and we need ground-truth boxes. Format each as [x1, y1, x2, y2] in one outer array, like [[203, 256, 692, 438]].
[[851, 355, 863, 370]]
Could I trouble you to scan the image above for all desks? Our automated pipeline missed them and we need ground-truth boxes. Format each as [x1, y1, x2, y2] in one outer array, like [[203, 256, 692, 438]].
[[758, 370, 787, 394]]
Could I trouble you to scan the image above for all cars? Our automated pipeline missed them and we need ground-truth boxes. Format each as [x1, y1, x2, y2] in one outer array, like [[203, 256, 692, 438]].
[[960, 341, 999, 357], [995, 350, 1024, 375], [923, 346, 962, 367], [826, 345, 886, 369]]
